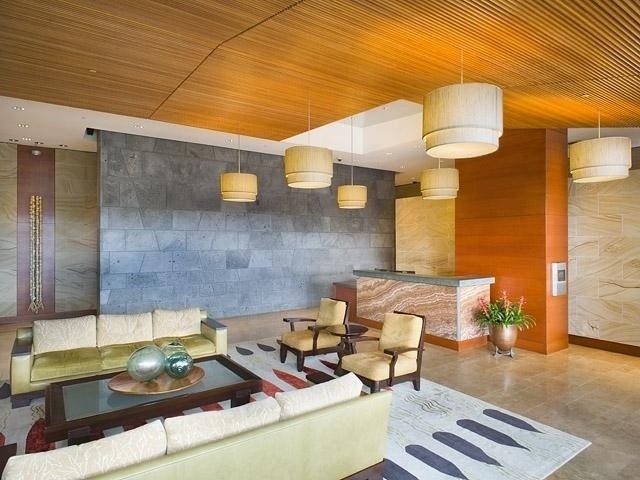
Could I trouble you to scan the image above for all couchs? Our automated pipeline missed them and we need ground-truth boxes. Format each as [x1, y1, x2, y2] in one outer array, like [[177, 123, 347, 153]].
[[2, 385, 393, 479], [10, 309, 227, 408]]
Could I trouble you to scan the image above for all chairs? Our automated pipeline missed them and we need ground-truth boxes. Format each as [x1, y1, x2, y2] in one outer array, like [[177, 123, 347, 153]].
[[341, 310, 426, 393], [276, 296, 349, 372]]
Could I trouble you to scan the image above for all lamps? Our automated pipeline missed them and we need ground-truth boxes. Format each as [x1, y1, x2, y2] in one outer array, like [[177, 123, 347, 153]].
[[423, 50, 505, 160], [283, 96, 334, 189], [420, 159, 459, 201], [221, 134, 257, 203], [337, 116, 367, 209], [569, 109, 632, 184]]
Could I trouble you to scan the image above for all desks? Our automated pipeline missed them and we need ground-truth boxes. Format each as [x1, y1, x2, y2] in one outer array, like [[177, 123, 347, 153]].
[[328, 326, 369, 375]]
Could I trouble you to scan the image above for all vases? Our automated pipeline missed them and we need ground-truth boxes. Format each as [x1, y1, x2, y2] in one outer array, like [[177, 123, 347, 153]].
[[488, 324, 520, 358]]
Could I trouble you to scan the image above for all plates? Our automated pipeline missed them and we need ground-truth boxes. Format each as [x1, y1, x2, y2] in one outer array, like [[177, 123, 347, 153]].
[[107, 365, 206, 396]]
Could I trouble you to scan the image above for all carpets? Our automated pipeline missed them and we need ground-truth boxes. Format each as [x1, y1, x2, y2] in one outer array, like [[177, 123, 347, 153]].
[[0, 334, 593, 479]]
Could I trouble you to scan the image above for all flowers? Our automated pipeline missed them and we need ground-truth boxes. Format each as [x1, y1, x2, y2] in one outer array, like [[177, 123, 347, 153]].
[[472, 288, 537, 331]]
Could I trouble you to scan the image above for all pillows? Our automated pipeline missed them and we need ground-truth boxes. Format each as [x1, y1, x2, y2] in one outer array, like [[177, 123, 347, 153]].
[[165, 395, 280, 455], [154, 307, 201, 340], [275, 372, 362, 421], [2, 418, 168, 476], [97, 312, 154, 347], [32, 314, 97, 354]]
